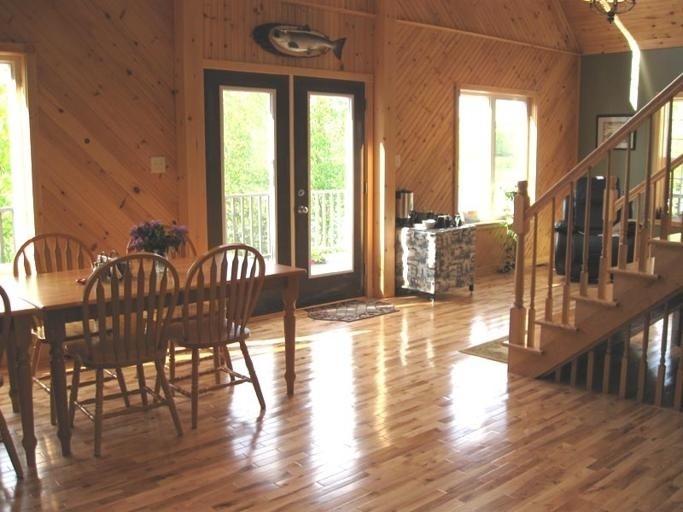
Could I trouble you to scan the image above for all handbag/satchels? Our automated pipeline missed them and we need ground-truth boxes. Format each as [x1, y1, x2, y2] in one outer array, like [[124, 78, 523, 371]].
[[92, 247, 129, 282]]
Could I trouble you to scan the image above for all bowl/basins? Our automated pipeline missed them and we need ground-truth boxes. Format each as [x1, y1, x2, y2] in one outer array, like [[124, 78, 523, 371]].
[[422, 219, 436, 228]]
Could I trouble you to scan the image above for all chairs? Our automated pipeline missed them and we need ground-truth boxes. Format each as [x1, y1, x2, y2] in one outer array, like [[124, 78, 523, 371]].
[[0, 286, 25, 481], [69, 254, 186, 458], [124, 226, 224, 382], [156, 242, 268, 430], [14, 234, 96, 431], [554, 176, 631, 283]]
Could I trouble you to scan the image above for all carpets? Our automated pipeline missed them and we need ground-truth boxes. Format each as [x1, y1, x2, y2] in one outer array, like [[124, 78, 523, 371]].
[[305, 299, 401, 325]]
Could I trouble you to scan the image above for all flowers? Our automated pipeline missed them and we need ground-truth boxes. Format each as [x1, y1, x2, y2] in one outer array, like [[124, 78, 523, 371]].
[[128, 219, 188, 251]]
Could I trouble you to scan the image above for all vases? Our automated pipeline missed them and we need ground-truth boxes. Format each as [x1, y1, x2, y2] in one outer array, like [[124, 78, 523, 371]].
[[154, 250, 170, 272]]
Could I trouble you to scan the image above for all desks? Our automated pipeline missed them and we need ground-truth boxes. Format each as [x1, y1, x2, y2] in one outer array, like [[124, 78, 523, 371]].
[[8, 296, 37, 467], [16, 250, 307, 457], [396, 223, 478, 304]]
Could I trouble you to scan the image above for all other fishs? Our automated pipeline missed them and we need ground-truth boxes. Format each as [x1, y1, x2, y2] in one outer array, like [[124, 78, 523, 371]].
[[271, 25, 347, 61]]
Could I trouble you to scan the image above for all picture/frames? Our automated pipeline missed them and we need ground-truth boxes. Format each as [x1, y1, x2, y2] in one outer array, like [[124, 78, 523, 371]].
[[596, 114, 635, 155]]
